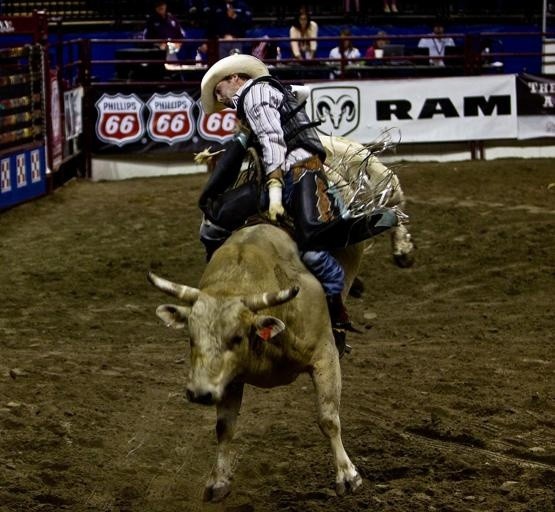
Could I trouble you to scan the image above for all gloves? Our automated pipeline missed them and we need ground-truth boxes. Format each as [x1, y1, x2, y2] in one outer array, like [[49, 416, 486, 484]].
[[267, 178, 284, 222]]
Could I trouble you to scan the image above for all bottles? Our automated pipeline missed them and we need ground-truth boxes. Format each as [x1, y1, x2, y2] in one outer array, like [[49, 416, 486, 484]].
[[195, 53, 202, 68]]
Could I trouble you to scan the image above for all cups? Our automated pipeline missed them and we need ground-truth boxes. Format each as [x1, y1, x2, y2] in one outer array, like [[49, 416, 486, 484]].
[[374, 48, 384, 58]]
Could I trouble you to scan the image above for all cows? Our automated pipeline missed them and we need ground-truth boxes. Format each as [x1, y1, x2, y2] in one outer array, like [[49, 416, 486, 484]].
[[146, 135, 417, 504]]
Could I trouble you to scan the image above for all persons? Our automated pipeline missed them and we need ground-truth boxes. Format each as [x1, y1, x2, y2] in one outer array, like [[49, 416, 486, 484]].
[[198, 53, 398, 334], [141, 0, 278, 60], [418, 20, 457, 67], [288, 5, 320, 60], [365, 30, 391, 60], [324, 28, 363, 80]]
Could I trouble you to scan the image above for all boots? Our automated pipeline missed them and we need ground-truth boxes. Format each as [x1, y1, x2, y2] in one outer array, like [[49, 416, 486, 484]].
[[328, 294, 349, 358]]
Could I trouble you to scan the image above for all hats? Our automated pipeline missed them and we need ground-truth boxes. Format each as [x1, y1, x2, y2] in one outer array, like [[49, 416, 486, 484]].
[[201, 55, 269, 113]]
